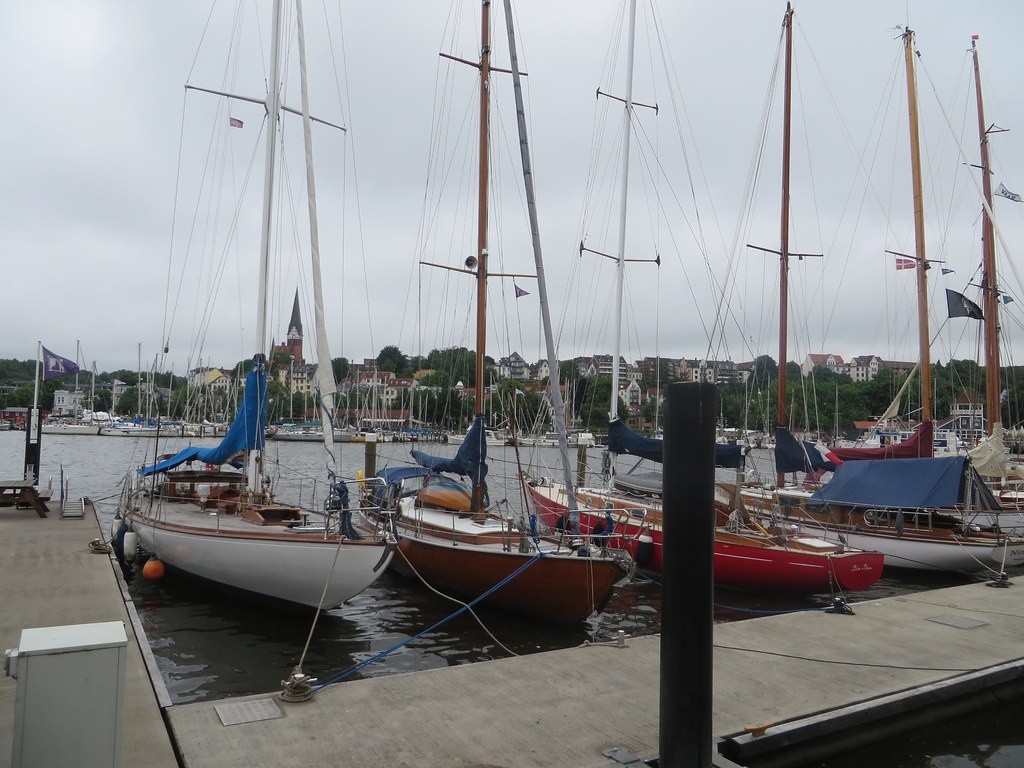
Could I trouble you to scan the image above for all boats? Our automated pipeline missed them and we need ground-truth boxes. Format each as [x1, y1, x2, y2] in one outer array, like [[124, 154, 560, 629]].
[[519, 426, 903, 449], [42, 417, 505, 447]]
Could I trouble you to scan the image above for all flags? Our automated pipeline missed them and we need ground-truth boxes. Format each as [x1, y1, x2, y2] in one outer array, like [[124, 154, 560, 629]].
[[992, 186, 1023, 203], [1003, 295, 1013, 305], [895, 258, 915, 271], [941, 268, 955, 276], [945, 289, 984, 319], [42, 345, 79, 381]]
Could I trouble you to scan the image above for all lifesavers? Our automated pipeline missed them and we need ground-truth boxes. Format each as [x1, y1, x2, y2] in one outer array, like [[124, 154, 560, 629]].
[[77, 405, 82, 410]]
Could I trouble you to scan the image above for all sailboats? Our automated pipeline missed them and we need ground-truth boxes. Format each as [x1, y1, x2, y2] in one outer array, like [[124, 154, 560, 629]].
[[112, 1, 398, 615], [525, 1, 888, 598], [616, 3, 1024, 576], [363, 2, 633, 635]]
